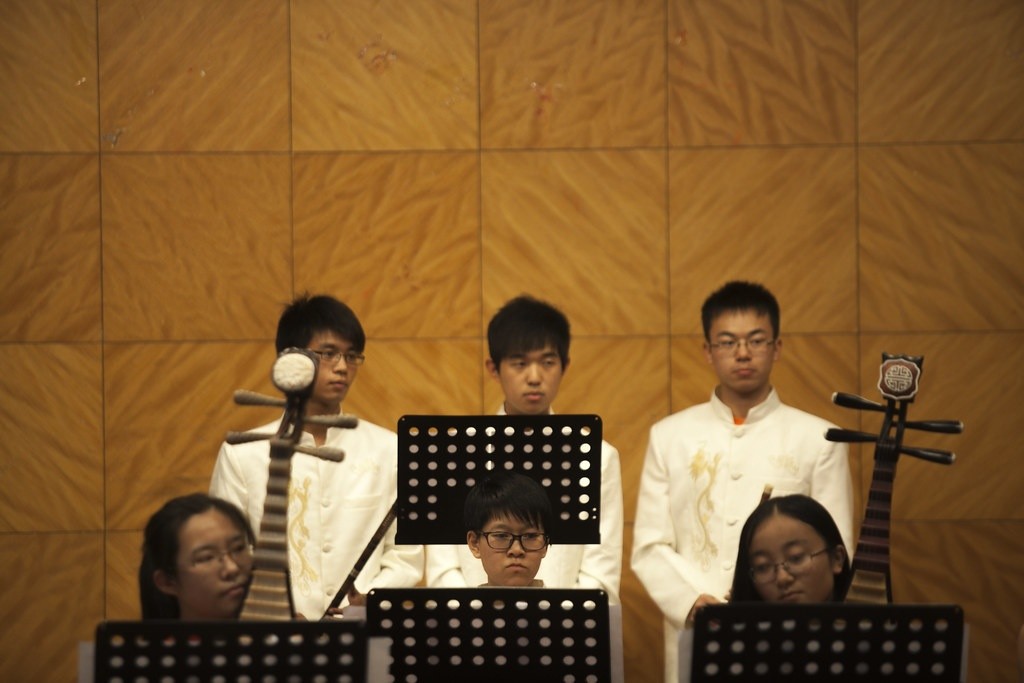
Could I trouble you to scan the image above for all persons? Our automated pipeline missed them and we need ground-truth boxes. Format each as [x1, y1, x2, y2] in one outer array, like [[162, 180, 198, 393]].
[[630, 279, 854, 683], [727, 493, 851, 605], [425, 292, 624, 683], [208, 289, 424, 621], [462, 468, 554, 608], [139, 490, 309, 643]]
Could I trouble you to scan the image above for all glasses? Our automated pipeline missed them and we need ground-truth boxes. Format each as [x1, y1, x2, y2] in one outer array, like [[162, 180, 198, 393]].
[[172, 536, 252, 582], [301, 347, 366, 366], [748, 547, 832, 586], [475, 528, 550, 552], [709, 336, 776, 355]]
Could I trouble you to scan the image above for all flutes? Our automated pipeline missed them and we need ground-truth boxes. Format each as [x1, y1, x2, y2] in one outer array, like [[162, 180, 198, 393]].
[[761, 484, 774, 503], [318, 499, 399, 620]]
